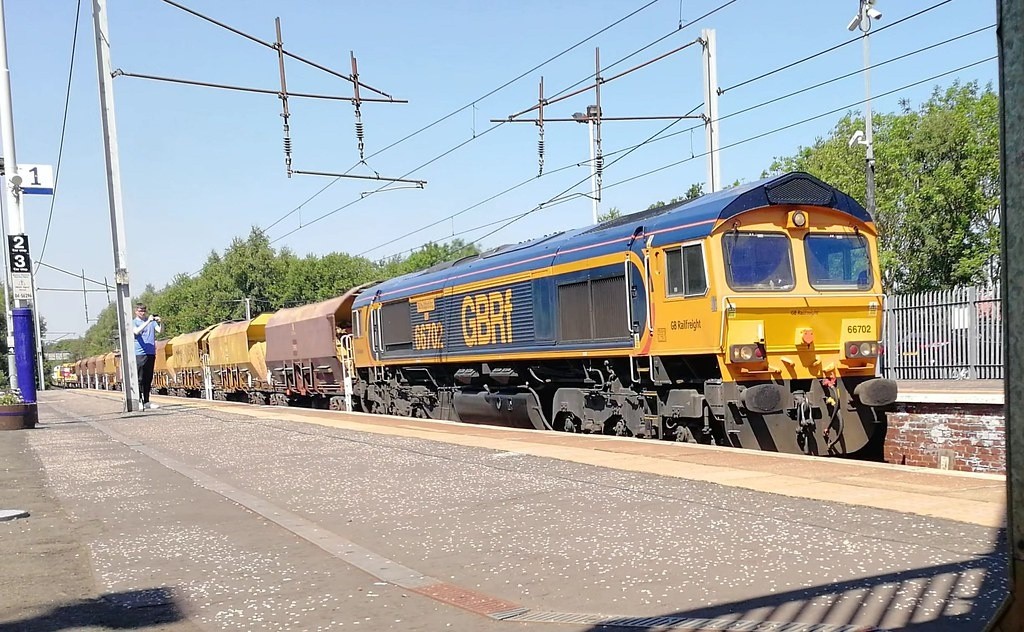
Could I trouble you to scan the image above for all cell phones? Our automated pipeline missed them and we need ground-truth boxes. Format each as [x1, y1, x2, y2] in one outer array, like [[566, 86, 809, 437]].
[[153, 313, 158, 321]]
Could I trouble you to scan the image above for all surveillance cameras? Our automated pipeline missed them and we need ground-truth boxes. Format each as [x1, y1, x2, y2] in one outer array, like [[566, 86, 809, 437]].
[[867, 9, 882, 19], [849, 131, 863, 147], [847, 15, 861, 31]]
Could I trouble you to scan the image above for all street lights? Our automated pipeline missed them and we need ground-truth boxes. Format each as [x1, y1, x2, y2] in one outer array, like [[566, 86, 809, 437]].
[[572, 104, 605, 223], [844, 0, 881, 225]]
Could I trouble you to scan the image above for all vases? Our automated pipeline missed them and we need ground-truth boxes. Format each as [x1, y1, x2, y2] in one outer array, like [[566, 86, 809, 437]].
[[0, 403, 35, 429]]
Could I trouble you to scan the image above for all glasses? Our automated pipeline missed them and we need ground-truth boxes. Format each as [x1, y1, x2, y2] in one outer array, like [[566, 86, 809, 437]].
[[137, 310, 146, 313]]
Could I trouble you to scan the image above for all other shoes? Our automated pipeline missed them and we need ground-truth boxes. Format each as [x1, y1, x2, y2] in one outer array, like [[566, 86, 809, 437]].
[[144, 402, 160, 409], [138, 401, 143, 411]]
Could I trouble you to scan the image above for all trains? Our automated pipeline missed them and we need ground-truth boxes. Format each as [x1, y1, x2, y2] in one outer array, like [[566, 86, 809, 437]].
[[54, 170, 902, 458]]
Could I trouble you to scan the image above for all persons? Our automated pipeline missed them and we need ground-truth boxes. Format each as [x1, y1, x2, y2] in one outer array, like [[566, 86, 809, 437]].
[[133, 303, 163, 409]]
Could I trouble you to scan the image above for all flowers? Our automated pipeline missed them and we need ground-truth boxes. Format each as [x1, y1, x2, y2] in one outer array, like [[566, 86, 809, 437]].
[[0, 388, 24, 404]]
